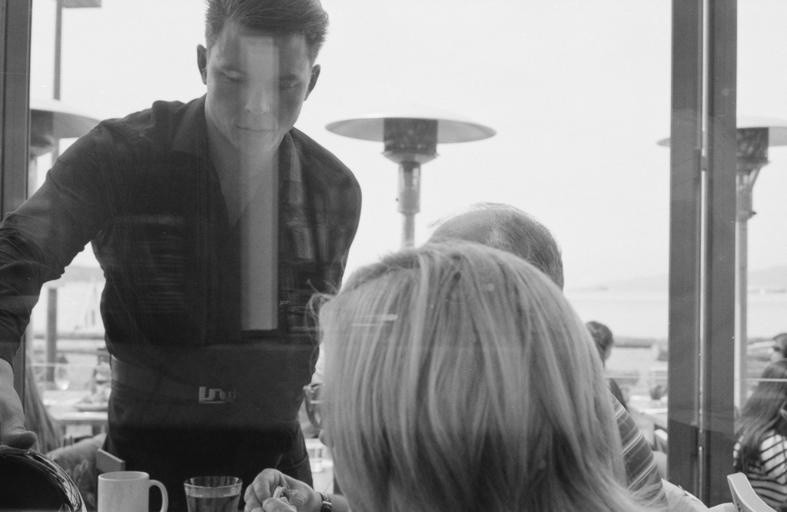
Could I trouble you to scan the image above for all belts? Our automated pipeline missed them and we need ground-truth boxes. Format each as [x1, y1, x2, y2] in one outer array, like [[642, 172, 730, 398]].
[[109, 353, 241, 406]]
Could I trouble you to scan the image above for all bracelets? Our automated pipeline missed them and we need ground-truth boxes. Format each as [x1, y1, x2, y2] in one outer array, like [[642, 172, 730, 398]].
[[318, 491, 333, 512]]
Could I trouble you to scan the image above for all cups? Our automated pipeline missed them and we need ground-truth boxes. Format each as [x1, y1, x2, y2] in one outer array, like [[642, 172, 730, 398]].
[[97, 471, 243, 512]]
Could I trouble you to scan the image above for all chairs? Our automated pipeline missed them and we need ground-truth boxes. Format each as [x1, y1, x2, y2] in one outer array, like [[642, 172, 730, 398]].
[[727, 472, 776, 512]]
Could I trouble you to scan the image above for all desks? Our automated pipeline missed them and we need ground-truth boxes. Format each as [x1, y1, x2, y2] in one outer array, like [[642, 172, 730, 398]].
[[44, 389, 108, 436], [627, 398, 668, 449]]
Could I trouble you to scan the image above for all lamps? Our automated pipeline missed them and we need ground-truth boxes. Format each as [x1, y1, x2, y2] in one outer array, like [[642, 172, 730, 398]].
[[656, 124, 787, 414], [29, 96, 102, 318], [325, 117, 496, 251]]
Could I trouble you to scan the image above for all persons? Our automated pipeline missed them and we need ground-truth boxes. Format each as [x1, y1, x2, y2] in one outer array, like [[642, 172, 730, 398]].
[[243, 235, 641, 511], [423, 199, 668, 511], [584, 313, 627, 410], [733, 361, 786, 512], [0, 0, 363, 512], [767, 330, 786, 360]]
